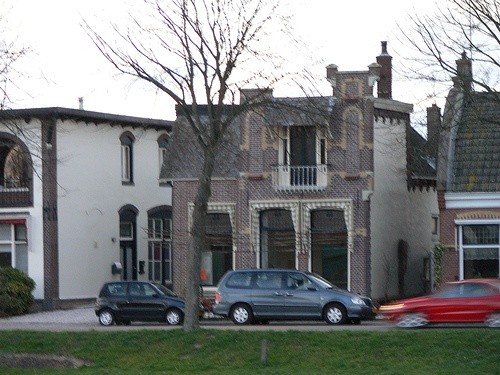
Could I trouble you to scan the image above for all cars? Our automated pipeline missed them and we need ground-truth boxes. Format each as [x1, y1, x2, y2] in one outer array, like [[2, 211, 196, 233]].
[[94, 281, 207, 326], [378, 278, 500, 328]]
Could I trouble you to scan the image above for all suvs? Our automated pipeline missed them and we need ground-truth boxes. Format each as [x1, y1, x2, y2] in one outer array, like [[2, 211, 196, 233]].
[[213, 268, 377, 326]]
